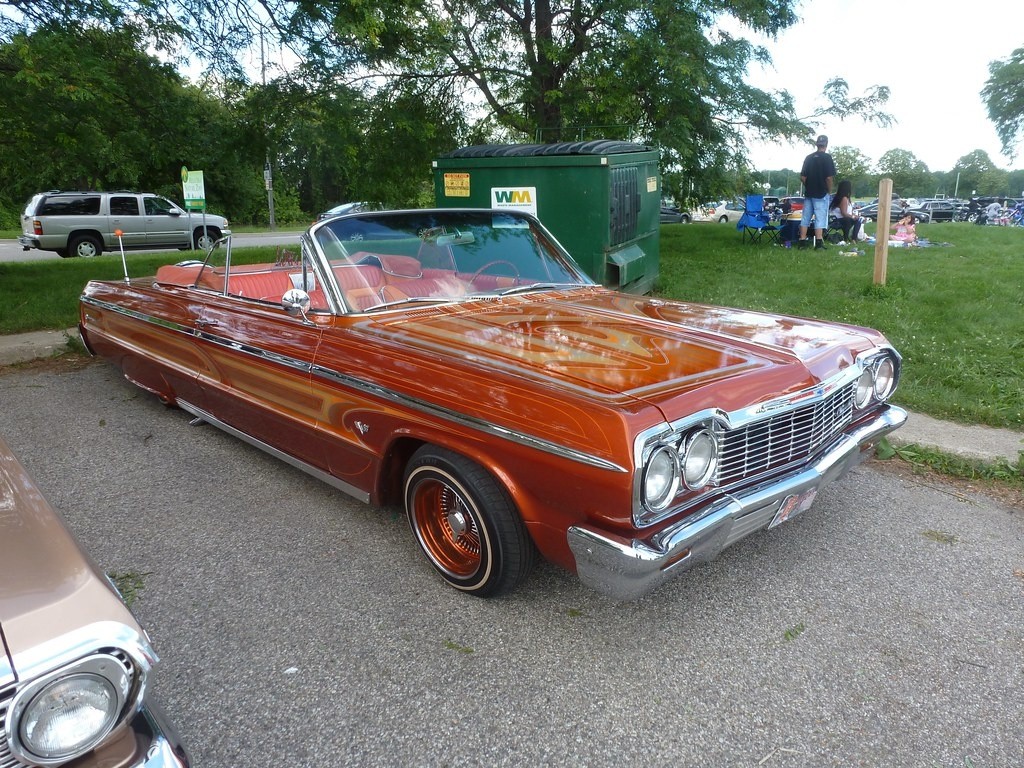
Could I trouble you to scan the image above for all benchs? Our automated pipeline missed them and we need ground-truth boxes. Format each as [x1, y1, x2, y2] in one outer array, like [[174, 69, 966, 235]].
[[260, 278, 452, 312], [226, 264, 385, 299]]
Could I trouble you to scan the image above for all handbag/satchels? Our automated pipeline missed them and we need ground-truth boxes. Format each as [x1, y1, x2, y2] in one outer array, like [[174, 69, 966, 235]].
[[848, 224, 864, 239]]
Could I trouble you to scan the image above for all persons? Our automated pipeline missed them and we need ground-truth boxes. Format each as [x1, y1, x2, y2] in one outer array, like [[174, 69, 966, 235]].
[[782, 200, 791, 214], [828, 181, 861, 244], [980, 197, 1001, 225], [889, 212, 918, 243], [797, 135, 836, 251], [965, 198, 977, 221]]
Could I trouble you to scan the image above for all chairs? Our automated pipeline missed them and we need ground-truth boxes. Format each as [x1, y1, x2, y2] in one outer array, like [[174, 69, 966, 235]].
[[737, 194, 787, 247]]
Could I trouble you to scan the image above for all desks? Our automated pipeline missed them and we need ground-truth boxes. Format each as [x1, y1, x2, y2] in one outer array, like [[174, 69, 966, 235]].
[[779, 217, 820, 246]]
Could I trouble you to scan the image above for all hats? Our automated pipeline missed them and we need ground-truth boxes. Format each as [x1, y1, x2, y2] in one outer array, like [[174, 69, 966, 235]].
[[815, 135, 828, 145]]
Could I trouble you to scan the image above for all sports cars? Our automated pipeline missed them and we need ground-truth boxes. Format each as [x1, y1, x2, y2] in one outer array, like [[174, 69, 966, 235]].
[[76, 208, 910, 599]]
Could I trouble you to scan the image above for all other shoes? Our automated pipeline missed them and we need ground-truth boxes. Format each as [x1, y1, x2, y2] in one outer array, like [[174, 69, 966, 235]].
[[815, 239, 827, 249], [798, 240, 809, 250]]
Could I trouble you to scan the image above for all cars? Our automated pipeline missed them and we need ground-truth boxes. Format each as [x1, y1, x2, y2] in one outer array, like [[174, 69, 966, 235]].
[[692, 201, 746, 224], [0, 437, 194, 768], [659, 206, 692, 224], [852, 194, 1024, 224], [780, 197, 806, 212], [318, 202, 422, 242]]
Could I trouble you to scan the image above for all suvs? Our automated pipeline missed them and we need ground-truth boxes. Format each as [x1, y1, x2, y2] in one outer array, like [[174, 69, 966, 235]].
[[16, 190, 232, 259]]
